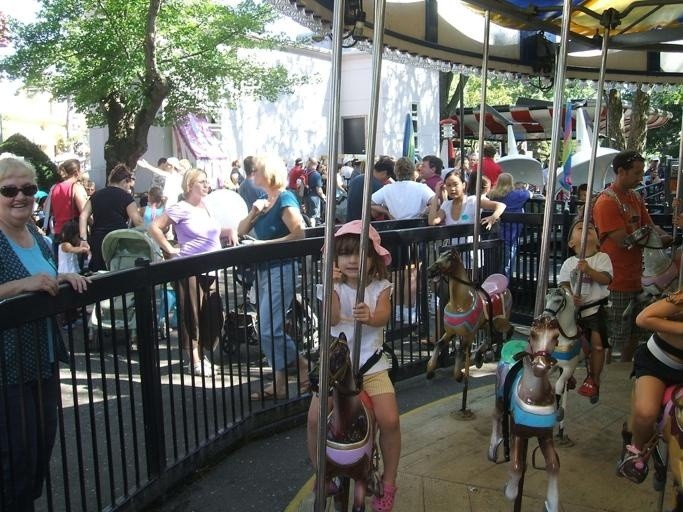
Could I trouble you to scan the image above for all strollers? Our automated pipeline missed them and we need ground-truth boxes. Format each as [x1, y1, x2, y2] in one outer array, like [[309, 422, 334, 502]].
[[88, 229, 167, 351], [221, 236, 319, 358]]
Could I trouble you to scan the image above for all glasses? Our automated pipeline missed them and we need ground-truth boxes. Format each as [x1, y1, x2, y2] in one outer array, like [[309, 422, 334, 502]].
[[1, 184, 38, 199], [625, 153, 642, 161]]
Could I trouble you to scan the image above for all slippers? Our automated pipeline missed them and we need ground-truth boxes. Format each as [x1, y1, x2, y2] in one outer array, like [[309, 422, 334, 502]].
[[300, 362, 313, 394], [251, 389, 286, 402], [313, 478, 337, 498], [370, 474, 398, 511]]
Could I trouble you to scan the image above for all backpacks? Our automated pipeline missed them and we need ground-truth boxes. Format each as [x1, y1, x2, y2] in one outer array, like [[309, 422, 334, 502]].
[[578, 193, 600, 223]]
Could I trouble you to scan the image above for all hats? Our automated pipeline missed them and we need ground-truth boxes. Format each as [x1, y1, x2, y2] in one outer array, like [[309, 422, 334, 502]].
[[319, 219, 391, 268]]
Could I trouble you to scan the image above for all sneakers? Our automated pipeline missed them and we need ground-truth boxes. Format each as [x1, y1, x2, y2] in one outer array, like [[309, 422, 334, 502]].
[[188, 361, 217, 377], [615, 442, 649, 484], [200, 355, 221, 370], [395, 304, 419, 324]]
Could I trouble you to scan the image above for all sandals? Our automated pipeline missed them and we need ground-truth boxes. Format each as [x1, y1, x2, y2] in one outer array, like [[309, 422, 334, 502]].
[[578, 376, 597, 396]]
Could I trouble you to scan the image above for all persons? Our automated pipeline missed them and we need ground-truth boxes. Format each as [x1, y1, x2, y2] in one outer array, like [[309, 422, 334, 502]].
[[1, 145, 683, 512]]
